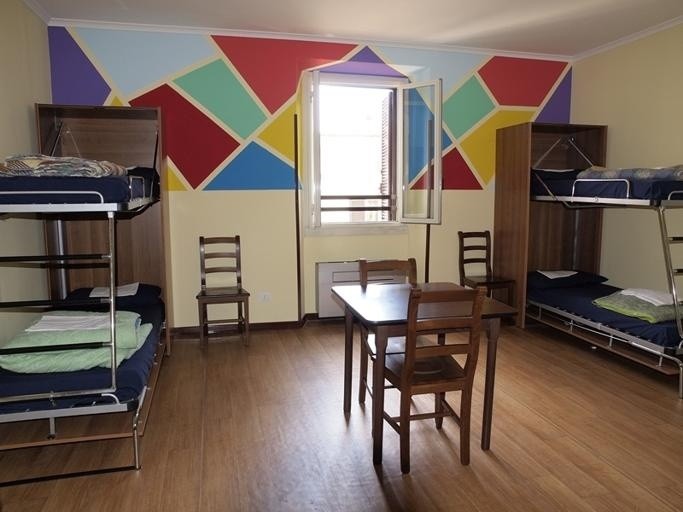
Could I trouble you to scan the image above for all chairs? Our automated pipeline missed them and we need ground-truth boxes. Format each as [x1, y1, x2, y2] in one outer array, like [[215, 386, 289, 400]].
[[368, 286, 487, 474], [358, 258, 417, 403], [458, 231, 515, 325], [197, 235, 251, 349]]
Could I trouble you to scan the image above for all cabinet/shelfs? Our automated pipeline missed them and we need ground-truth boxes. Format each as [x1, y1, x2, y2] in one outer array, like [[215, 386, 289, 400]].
[[35, 102, 174, 355], [492, 121, 608, 330]]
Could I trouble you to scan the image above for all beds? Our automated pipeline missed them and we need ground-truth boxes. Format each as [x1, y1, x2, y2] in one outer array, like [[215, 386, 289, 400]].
[[0, 158, 166, 486], [524, 166, 683, 398]]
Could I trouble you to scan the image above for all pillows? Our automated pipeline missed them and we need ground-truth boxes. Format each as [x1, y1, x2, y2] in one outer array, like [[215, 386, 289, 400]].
[[125, 166, 159, 182], [527, 269, 610, 293], [533, 168, 582, 179], [60, 283, 161, 308]]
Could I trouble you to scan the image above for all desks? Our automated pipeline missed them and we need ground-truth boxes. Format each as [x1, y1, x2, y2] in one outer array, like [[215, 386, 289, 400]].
[[330, 282, 520, 465]]
